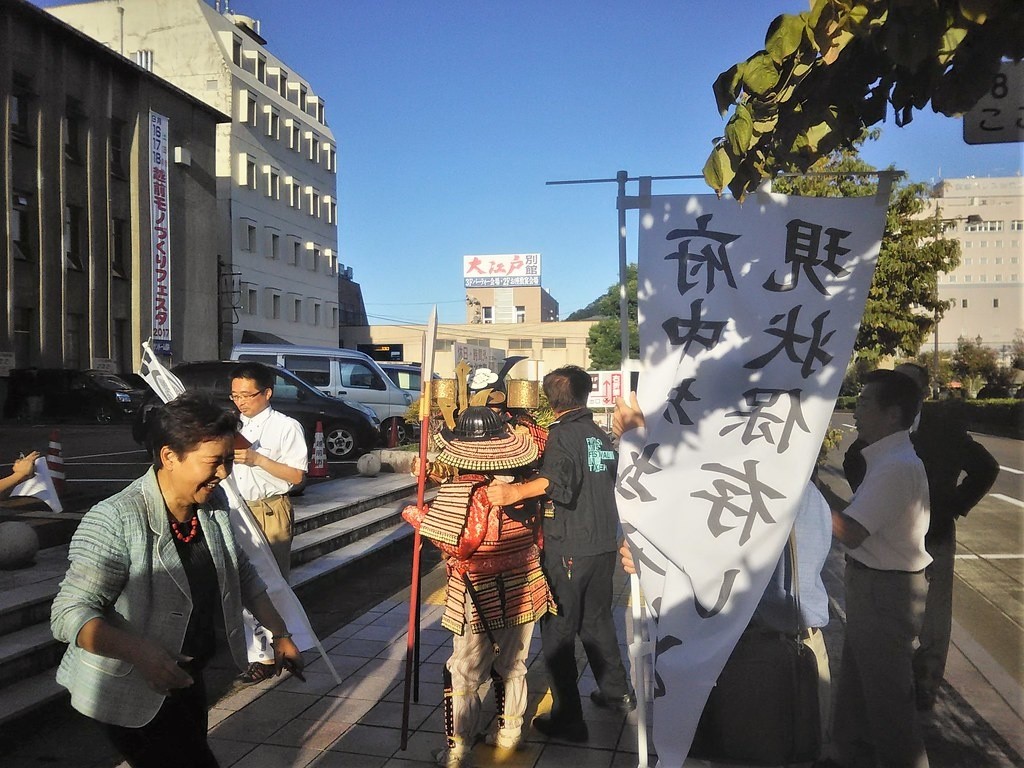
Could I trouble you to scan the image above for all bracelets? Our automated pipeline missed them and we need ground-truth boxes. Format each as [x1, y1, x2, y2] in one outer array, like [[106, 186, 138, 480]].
[[271, 632, 293, 638]]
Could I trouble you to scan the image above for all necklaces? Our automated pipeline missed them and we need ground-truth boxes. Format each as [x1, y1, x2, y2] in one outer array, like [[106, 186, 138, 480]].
[[168, 507, 199, 542]]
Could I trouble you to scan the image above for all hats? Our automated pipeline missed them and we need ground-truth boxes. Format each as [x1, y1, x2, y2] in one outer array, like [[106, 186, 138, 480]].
[[433, 413, 538, 471]]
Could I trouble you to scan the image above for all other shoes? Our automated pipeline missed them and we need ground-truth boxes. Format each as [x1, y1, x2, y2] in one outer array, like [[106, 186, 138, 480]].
[[591, 690, 637, 713], [234, 662, 274, 686], [533, 718, 589, 743], [476, 731, 525, 751], [917, 699, 945, 728], [431, 746, 473, 768]]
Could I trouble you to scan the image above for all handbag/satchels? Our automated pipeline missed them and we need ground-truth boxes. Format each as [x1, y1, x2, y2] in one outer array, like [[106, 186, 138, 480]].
[[686, 637, 821, 764]]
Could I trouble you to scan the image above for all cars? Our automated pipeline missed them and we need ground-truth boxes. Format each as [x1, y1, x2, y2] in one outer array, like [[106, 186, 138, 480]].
[[14, 368, 147, 424], [342, 359, 445, 401], [116, 372, 157, 397], [131, 360, 381, 457]]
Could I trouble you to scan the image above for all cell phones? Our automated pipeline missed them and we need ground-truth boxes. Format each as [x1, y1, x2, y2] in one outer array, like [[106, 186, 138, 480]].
[[270, 643, 306, 683]]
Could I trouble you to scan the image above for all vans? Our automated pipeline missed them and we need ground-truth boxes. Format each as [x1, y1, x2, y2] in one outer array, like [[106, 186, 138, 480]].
[[229, 343, 415, 446]]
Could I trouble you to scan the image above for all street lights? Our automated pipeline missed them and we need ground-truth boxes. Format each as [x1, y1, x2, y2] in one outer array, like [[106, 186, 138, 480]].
[[933, 214, 984, 398]]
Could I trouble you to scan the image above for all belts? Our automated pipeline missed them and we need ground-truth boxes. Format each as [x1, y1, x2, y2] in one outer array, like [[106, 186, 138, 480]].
[[843, 554, 924, 575]]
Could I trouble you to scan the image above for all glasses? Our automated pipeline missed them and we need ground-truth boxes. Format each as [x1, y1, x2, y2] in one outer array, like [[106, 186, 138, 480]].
[[228, 387, 267, 401], [854, 396, 880, 411]]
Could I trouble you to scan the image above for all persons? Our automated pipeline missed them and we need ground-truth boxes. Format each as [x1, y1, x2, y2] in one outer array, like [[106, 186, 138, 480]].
[[51, 389, 305, 768], [487, 365, 638, 744], [0, 450, 40, 501], [402, 354, 560, 768], [612, 361, 999, 768], [228, 363, 308, 687]]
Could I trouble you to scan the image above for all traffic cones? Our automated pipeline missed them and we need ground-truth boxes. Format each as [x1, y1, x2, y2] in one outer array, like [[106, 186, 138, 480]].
[[390, 417, 400, 448], [42, 431, 75, 500], [303, 421, 336, 477]]
